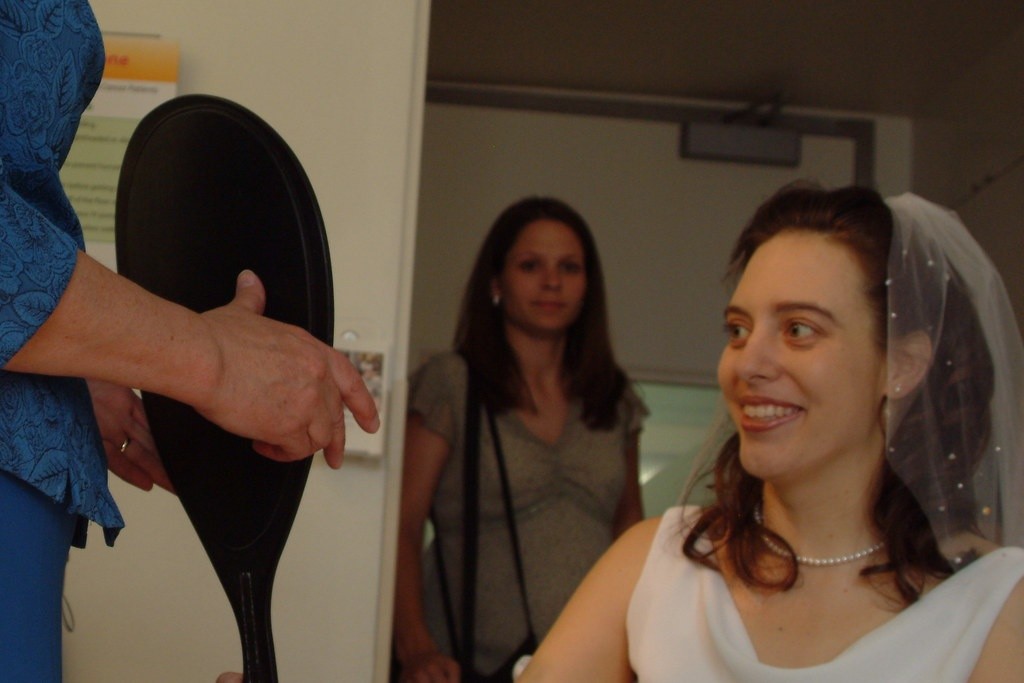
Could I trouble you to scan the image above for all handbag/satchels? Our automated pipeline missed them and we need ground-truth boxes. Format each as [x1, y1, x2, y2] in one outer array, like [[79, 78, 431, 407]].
[[469, 634, 546, 683]]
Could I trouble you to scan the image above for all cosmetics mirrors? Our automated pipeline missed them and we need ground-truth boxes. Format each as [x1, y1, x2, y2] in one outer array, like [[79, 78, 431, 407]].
[[116, 93, 334, 683]]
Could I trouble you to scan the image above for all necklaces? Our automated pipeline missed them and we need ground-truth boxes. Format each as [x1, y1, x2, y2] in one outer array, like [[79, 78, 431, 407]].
[[751, 508, 884, 564]]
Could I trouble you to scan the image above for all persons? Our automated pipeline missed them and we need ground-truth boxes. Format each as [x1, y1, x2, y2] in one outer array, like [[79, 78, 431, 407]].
[[393, 195, 651, 683], [0, 0, 382, 683], [213, 182, 1024, 683]]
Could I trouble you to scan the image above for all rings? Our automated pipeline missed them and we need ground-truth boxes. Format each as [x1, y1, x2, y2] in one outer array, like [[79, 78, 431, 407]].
[[120, 438, 130, 452]]
